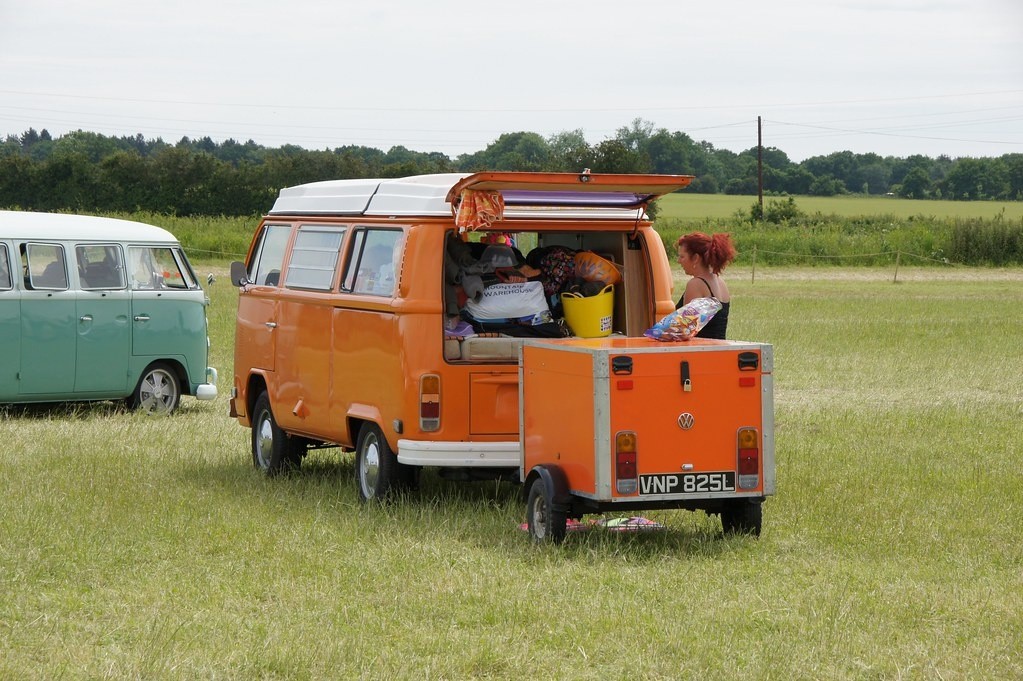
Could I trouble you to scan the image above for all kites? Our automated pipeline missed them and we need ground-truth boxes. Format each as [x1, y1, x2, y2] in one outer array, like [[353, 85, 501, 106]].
[[519, 516, 666, 534]]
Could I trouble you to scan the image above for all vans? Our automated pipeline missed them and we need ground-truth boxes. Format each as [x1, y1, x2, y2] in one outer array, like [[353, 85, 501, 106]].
[[228, 170, 695, 506], [0, 210, 219, 420]]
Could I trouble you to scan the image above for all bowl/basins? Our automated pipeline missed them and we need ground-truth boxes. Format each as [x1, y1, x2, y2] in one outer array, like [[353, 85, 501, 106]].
[[479, 244, 519, 268], [575, 251, 621, 286]]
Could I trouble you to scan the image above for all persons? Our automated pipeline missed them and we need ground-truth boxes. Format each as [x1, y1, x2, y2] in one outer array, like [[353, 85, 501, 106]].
[[673, 232, 736, 341], [41, 247, 83, 278]]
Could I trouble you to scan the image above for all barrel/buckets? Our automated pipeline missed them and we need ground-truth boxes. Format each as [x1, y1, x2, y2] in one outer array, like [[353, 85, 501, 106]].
[[561, 284, 615, 338]]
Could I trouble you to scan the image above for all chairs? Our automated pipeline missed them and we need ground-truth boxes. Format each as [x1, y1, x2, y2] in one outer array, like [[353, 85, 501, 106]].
[[84, 262, 120, 288]]
